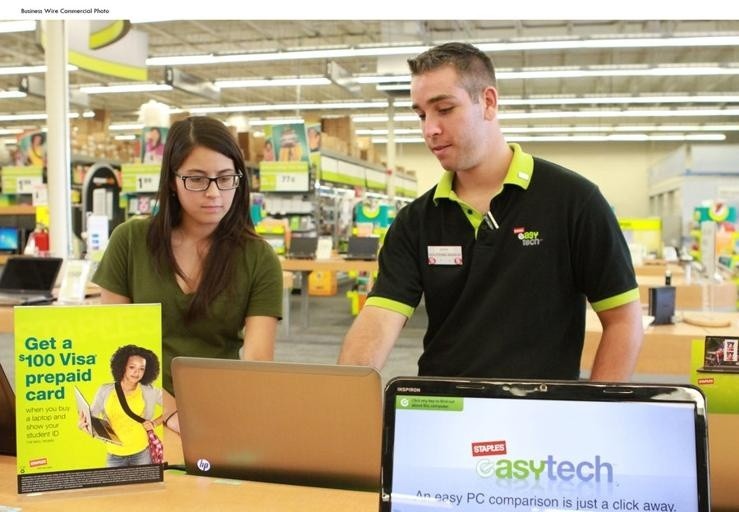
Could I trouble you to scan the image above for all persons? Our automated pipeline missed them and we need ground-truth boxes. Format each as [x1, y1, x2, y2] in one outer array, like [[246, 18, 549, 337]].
[[75, 345, 164, 467], [262, 140, 273, 159], [145, 129, 163, 164], [336, 41, 646, 383], [89, 117, 284, 438], [27, 132, 44, 167]]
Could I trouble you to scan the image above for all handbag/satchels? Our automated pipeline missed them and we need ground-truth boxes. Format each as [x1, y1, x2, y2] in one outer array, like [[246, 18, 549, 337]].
[[148, 429, 164, 465]]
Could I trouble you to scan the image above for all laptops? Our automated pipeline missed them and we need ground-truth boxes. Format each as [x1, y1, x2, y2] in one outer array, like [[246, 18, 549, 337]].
[[284, 237, 318, 259], [381, 376, 710, 511], [170, 354, 383, 494], [1, 257, 63, 305], [343, 236, 379, 261]]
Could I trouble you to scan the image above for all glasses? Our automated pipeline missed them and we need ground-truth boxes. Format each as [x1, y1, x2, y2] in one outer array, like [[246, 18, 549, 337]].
[[174, 168, 243, 192]]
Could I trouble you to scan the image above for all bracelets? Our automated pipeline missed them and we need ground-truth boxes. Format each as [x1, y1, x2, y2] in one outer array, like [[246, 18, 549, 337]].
[[165, 409, 177, 426]]
